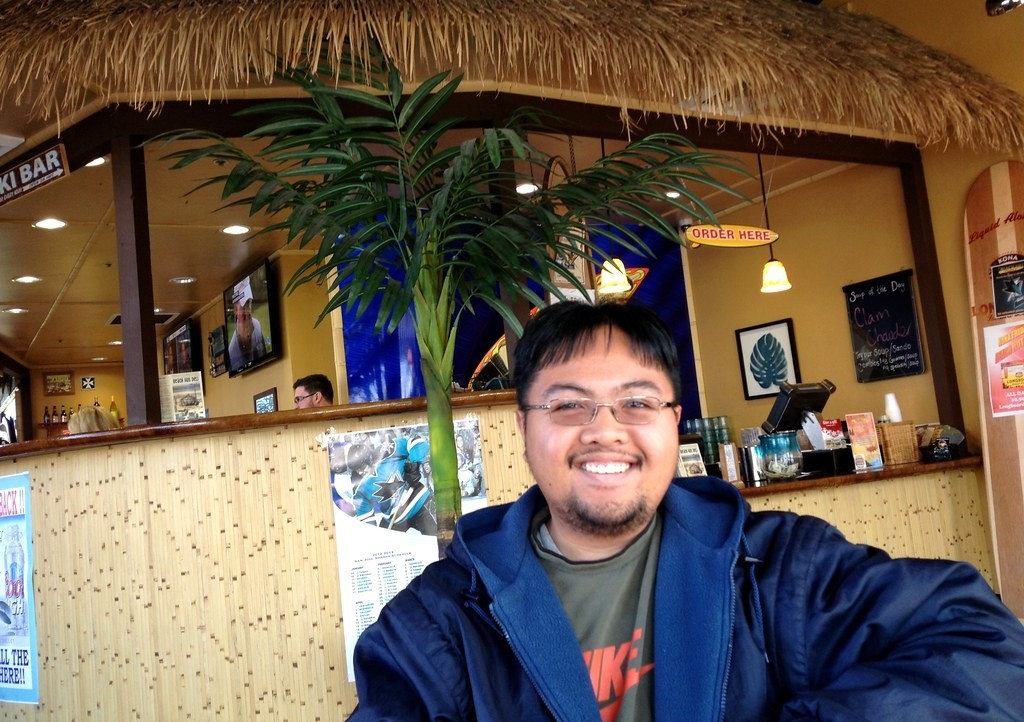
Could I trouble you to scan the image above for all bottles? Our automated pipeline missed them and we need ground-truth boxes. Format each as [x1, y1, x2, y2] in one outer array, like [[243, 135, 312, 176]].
[[69, 407, 74, 419], [52, 406, 59, 423], [4, 525, 25, 631], [78, 404, 81, 411], [110, 396, 118, 420], [44, 406, 50, 423], [61, 405, 67, 422], [94, 395, 100, 406]]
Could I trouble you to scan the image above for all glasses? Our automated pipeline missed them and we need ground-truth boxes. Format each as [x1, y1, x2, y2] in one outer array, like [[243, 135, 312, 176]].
[[294, 392, 316, 404], [521, 396, 678, 427]]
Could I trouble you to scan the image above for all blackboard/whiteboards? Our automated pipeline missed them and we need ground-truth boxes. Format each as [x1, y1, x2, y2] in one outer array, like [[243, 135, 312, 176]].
[[841, 267, 926, 384]]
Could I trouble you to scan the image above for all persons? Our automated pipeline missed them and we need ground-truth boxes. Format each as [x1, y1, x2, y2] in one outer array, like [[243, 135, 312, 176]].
[[64, 406, 116, 435], [346, 298, 1023, 722], [165, 339, 193, 374], [228, 276, 268, 371], [293, 374, 334, 410]]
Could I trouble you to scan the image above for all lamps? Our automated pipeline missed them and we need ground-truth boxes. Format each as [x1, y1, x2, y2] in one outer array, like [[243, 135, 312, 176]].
[[597, 137, 633, 294], [755, 153, 793, 295]]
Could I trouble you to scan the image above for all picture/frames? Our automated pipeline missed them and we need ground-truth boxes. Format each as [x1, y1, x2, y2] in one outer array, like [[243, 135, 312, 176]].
[[733, 317, 802, 401], [253, 387, 279, 415], [43, 370, 75, 396]]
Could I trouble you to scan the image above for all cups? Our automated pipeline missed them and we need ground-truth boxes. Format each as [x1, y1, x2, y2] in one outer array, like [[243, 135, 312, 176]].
[[686, 415, 731, 463], [738, 446, 766, 483], [759, 431, 803, 480], [885, 393, 902, 423]]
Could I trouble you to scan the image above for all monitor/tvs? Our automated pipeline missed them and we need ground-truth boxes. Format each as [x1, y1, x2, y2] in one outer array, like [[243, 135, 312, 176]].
[[162, 318, 206, 394], [223, 258, 283, 378]]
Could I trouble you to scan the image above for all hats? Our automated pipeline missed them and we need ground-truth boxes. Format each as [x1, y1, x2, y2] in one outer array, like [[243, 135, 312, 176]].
[[234, 276, 254, 306]]
